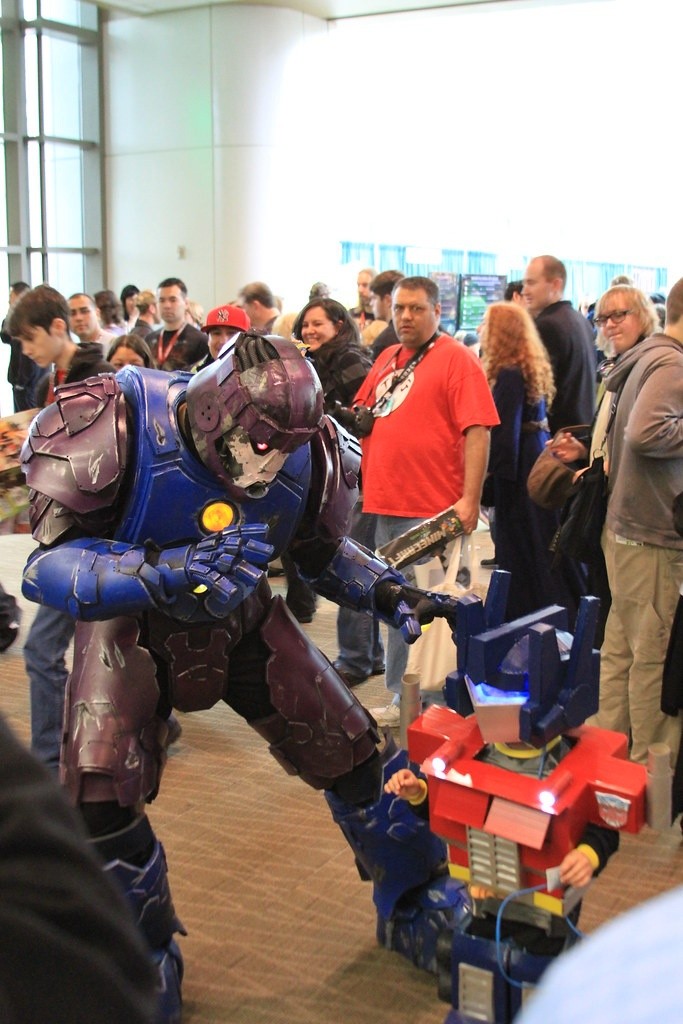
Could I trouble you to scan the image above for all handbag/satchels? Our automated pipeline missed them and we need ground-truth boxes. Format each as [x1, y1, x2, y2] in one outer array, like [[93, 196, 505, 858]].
[[549, 456, 605, 578], [527, 425, 594, 513]]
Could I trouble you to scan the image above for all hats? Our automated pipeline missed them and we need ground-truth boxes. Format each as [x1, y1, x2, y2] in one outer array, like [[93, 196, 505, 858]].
[[311, 281, 331, 297], [200, 305, 251, 332], [135, 292, 156, 306]]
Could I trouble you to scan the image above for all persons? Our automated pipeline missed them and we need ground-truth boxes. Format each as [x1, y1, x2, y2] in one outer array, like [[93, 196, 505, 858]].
[[0, 256, 683, 1024], [384, 570, 649, 1024], [20, 331, 471, 1024]]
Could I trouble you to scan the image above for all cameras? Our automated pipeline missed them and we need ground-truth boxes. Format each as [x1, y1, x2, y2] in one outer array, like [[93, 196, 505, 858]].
[[327, 400, 375, 440]]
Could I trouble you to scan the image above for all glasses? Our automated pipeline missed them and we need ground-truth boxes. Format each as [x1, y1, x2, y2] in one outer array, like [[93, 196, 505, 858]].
[[593, 308, 634, 327]]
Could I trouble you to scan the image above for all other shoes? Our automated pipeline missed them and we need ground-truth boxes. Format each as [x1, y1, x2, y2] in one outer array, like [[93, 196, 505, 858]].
[[0, 606, 22, 652], [456, 568, 472, 591], [144, 714, 181, 804], [287, 606, 313, 623], [478, 505, 490, 525], [332, 656, 385, 688], [266, 566, 285, 578], [480, 559, 499, 569], [369, 704, 422, 727]]
